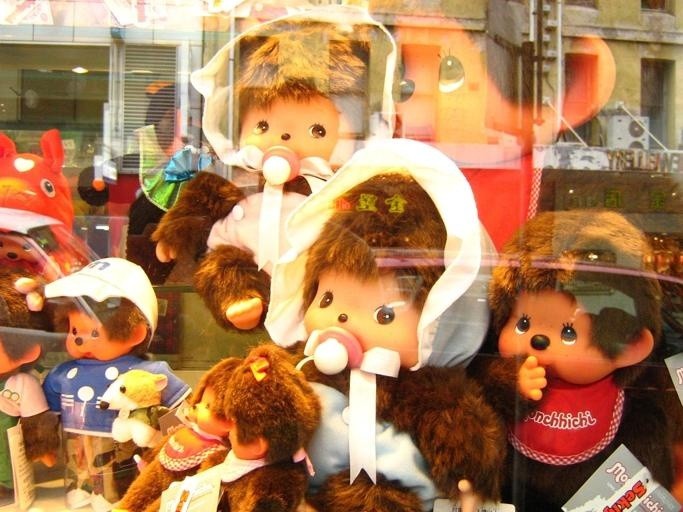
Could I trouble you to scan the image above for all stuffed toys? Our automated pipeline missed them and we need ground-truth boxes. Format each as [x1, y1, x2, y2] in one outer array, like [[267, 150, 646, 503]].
[[0, 0, 682, 512], [2, 0, 683, 512]]
